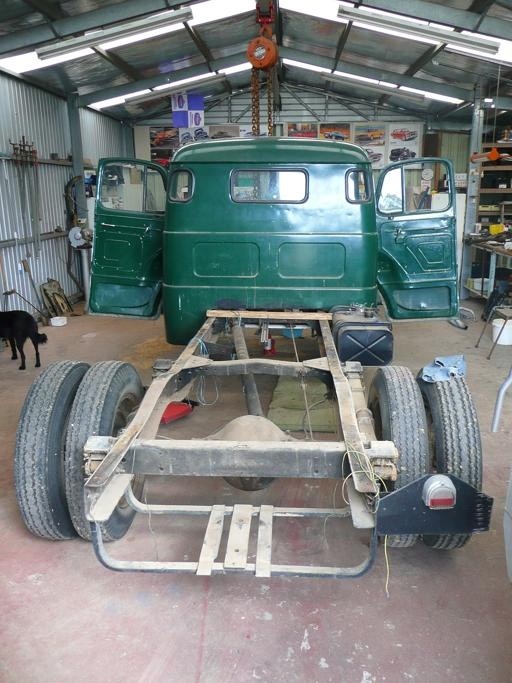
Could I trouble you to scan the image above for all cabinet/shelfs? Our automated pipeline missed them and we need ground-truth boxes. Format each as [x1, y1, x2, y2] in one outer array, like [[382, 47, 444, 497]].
[[476, 142, 512, 232]]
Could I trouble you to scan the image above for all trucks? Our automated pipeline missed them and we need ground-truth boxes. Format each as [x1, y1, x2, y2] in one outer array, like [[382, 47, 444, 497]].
[[13, 135, 495, 579]]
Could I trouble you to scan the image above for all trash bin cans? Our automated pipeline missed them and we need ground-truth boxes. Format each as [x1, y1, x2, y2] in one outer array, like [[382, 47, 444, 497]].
[[492, 318, 512, 345]]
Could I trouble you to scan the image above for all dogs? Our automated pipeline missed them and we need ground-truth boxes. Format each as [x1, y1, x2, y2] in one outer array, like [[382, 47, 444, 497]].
[[0, 310, 48, 370]]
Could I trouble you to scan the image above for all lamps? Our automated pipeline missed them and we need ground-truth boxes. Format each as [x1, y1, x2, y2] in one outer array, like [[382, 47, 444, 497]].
[[336, 4, 501, 54], [36, 5, 194, 60], [470, 63, 501, 162]]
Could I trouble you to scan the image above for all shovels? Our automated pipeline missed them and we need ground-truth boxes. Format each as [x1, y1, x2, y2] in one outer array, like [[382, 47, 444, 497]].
[[26, 259, 49, 325]]
